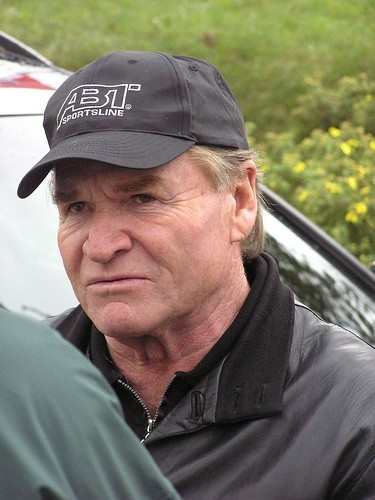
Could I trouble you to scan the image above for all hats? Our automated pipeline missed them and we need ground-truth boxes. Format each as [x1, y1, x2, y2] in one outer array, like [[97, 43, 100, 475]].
[[16, 49, 250, 201]]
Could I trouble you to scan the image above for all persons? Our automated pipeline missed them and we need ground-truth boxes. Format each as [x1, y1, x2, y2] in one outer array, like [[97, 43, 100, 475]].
[[0, 305, 181, 500], [17, 50, 375, 500]]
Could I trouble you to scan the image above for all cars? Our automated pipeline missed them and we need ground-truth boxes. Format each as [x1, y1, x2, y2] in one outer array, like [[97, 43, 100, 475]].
[[0, 28, 375, 358]]
